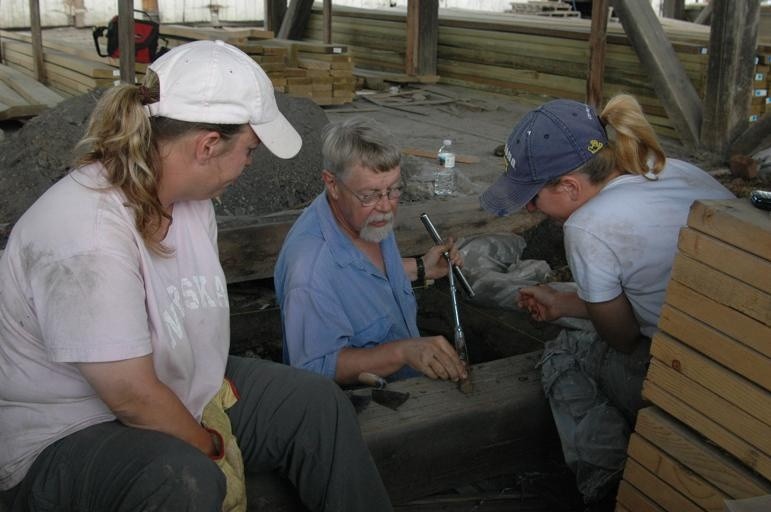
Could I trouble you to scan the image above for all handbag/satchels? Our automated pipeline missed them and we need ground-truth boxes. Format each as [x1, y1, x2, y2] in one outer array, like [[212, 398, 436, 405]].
[[106, 15, 157, 64]]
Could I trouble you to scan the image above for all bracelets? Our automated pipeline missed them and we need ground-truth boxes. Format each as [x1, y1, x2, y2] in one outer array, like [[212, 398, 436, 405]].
[[415, 257, 426, 282]]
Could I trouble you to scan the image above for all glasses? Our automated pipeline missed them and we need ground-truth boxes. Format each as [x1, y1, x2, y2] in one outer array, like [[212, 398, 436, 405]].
[[335, 171, 407, 208]]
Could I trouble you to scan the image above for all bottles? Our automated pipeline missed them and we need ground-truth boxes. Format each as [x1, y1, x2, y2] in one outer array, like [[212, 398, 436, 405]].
[[434, 138, 456, 196]]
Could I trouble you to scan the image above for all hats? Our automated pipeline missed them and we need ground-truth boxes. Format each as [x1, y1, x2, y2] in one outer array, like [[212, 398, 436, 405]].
[[477, 98, 609, 219], [139, 38, 303, 161]]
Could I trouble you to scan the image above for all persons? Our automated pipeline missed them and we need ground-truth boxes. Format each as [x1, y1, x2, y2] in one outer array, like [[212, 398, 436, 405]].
[[266, 115, 470, 389], [0, 38, 397, 511], [477, 93, 744, 511]]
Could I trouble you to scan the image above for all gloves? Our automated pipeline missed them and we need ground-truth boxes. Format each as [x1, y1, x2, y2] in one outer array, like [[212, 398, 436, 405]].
[[200, 376, 248, 507]]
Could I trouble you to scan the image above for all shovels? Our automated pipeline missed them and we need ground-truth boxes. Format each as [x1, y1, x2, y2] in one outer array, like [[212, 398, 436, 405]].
[[359, 372, 409, 410]]
